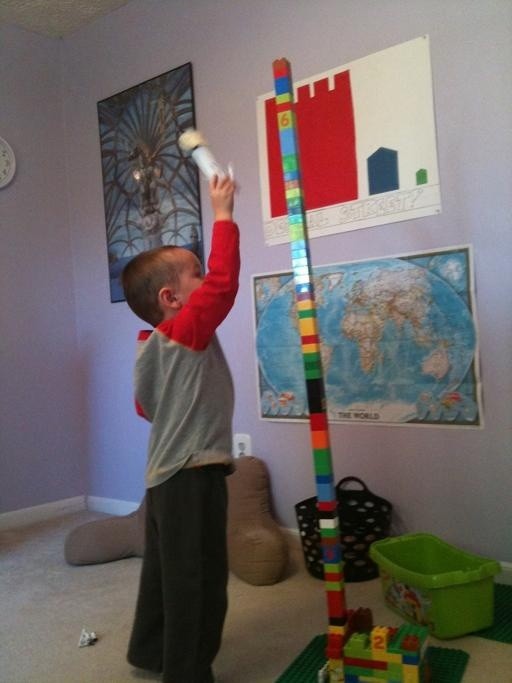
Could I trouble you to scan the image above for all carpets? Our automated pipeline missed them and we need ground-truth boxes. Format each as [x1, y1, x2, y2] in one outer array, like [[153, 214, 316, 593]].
[[470, 581, 511, 643], [274, 635, 470, 682]]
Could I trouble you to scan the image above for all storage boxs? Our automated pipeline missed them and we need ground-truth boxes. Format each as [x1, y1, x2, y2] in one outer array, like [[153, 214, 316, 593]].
[[369, 531, 501, 639]]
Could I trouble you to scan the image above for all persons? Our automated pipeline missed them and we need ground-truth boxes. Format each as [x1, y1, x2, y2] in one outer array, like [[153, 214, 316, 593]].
[[119, 170, 241, 681]]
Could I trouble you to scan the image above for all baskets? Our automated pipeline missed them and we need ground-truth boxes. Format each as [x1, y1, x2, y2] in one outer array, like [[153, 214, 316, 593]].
[[294, 476, 392, 583]]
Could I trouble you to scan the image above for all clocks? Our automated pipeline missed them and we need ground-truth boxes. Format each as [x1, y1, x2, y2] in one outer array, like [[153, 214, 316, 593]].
[[1, 138, 16, 190]]
